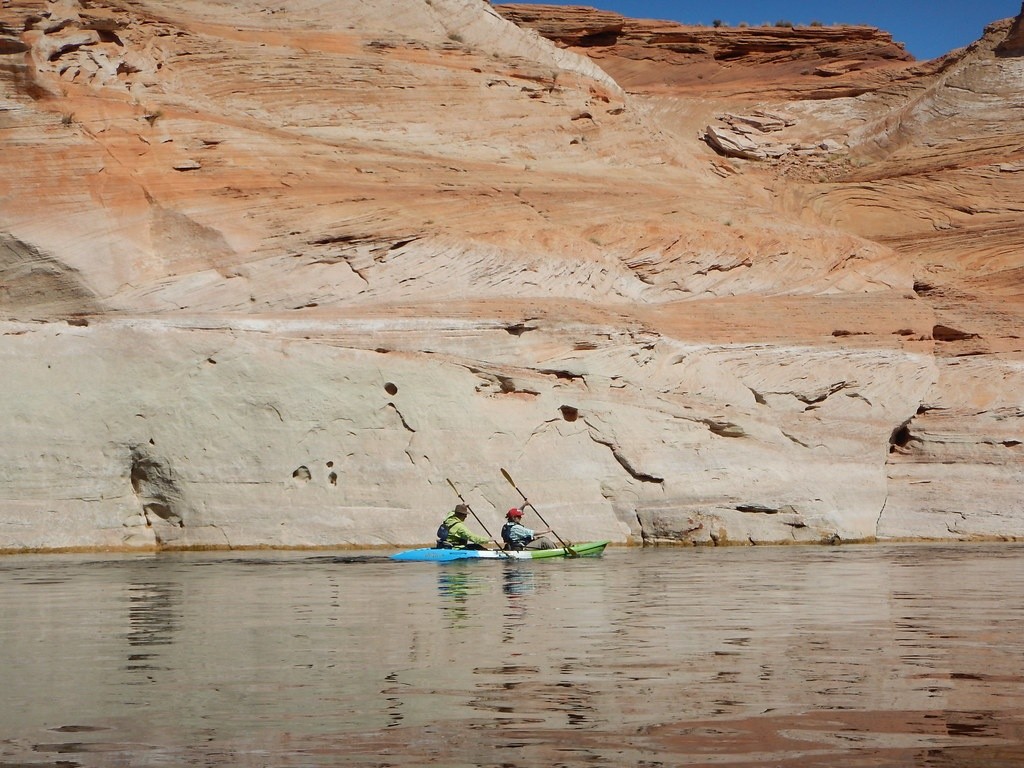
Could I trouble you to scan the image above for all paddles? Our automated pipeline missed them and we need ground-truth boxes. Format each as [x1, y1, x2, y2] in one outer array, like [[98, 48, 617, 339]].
[[445, 476, 513, 558], [499, 467, 579, 557]]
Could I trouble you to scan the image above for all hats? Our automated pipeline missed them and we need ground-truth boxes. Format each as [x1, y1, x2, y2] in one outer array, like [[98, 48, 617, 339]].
[[507, 508, 525, 518], [452, 504, 471, 514]]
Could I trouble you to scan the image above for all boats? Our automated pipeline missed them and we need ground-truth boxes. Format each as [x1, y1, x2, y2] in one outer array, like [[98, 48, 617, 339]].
[[387, 540, 611, 562]]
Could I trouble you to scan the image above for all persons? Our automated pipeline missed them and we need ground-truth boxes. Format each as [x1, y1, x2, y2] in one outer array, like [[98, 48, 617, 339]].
[[438, 503, 497, 550], [499, 498, 557, 550]]
